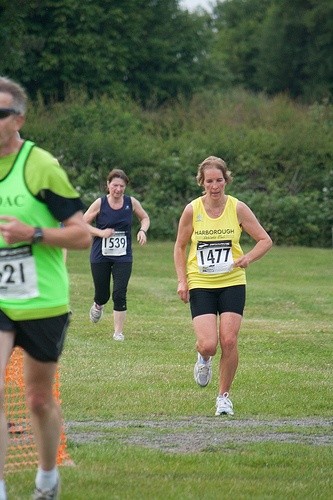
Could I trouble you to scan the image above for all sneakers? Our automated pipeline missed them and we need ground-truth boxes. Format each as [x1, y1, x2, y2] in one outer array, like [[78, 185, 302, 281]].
[[194, 352, 212, 386], [90, 302, 103, 325], [214, 391, 234, 415], [113, 332, 124, 341]]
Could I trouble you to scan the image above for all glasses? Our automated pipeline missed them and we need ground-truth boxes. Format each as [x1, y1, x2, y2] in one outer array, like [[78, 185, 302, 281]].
[[0, 107, 21, 119]]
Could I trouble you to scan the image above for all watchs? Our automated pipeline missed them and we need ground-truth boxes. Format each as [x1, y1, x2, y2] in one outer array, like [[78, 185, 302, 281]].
[[30, 227, 43, 244]]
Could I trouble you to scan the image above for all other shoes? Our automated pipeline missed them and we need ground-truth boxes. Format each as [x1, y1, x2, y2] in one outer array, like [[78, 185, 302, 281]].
[[33, 476, 59, 500]]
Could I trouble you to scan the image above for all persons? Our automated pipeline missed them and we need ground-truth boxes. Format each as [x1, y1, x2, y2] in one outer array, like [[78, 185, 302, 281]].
[[0, 77, 91, 500], [174, 156, 272, 412], [80, 169, 149, 341]]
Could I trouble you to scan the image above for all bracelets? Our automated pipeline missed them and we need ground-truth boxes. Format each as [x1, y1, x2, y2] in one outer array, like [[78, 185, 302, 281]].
[[139, 230, 146, 235]]
